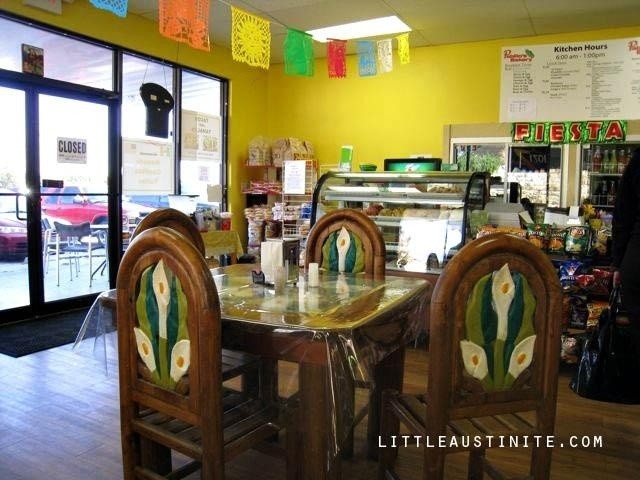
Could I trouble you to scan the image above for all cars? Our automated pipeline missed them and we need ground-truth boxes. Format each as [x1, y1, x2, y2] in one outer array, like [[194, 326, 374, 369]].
[[0, 187, 57, 262], [89, 192, 161, 226]]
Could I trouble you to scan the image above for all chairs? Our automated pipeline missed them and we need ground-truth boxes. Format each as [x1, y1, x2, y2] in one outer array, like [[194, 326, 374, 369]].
[[304, 209, 386, 277], [377, 232, 563, 480], [129, 208, 280, 445], [115, 227, 293, 480], [41, 217, 108, 287]]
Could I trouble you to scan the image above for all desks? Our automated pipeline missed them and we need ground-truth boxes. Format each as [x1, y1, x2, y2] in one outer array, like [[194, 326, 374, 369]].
[[201, 230, 244, 264]]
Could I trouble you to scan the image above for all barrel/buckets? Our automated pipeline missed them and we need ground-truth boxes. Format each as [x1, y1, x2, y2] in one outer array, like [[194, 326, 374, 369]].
[[168, 195, 197, 218]]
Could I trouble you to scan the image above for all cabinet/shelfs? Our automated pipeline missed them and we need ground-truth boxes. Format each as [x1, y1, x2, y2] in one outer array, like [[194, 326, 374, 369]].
[[240, 153, 317, 239], [309, 170, 490, 282]]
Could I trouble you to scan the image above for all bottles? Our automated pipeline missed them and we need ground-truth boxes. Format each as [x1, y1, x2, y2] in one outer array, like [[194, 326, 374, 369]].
[[589, 146, 632, 206]]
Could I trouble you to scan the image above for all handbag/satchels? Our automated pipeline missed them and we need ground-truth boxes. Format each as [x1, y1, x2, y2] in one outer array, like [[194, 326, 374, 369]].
[[570, 284, 640, 405]]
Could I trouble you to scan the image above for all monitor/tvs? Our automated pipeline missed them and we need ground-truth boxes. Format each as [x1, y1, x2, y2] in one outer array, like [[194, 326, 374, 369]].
[[384, 158, 442, 171]]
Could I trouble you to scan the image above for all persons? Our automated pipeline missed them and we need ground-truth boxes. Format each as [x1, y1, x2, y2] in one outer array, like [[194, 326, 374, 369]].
[[611, 145, 639, 402]]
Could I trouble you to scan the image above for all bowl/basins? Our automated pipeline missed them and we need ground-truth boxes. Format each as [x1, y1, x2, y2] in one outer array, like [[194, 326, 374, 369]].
[[359, 164, 377, 171]]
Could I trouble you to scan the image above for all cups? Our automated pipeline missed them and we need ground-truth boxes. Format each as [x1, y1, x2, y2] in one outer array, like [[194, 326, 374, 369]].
[[273, 266, 286, 291]]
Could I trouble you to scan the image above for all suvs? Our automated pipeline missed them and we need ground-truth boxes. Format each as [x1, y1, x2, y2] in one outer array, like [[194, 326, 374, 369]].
[[39, 181, 160, 250], [128, 192, 220, 224]]
